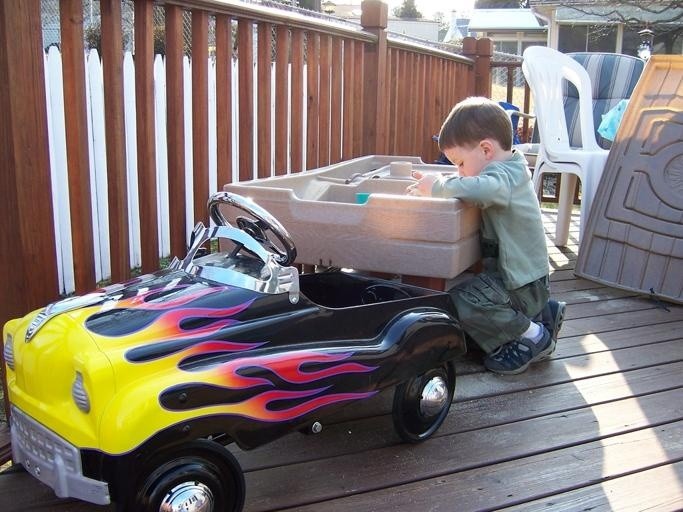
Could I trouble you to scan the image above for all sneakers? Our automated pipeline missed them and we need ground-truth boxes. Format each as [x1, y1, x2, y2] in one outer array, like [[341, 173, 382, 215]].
[[483, 322, 555, 374], [543, 299, 566, 341]]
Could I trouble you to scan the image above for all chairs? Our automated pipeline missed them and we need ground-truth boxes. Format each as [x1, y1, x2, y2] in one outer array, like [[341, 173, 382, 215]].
[[507, 43, 646, 247]]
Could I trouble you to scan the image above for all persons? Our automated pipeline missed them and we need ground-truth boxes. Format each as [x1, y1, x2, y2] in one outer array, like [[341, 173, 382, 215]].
[[407, 95, 567, 374]]
